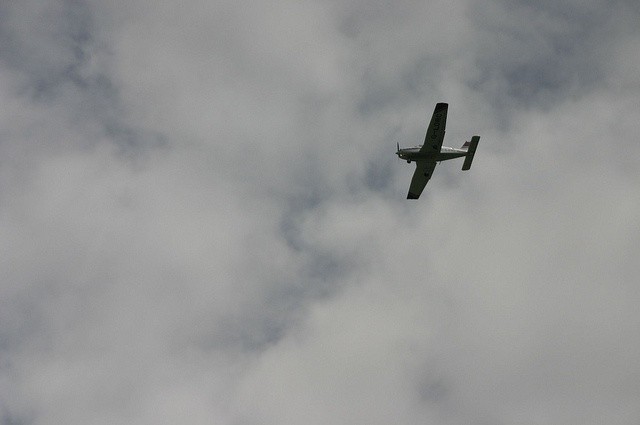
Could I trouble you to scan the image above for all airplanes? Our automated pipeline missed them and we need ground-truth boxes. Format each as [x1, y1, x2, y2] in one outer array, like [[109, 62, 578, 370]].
[[395, 103, 480, 199]]
